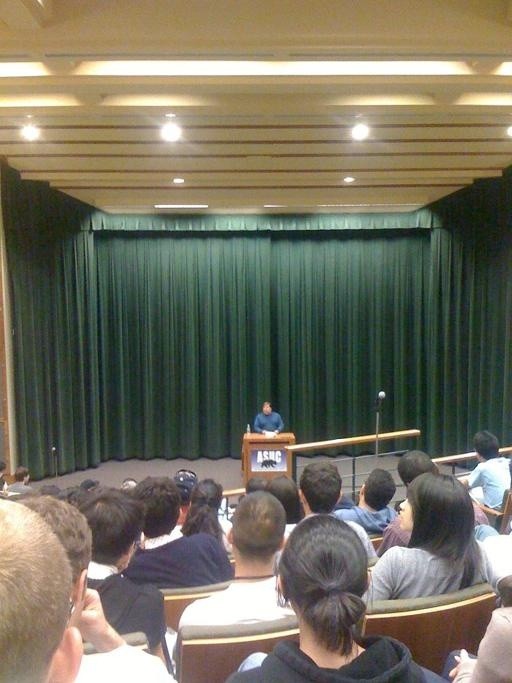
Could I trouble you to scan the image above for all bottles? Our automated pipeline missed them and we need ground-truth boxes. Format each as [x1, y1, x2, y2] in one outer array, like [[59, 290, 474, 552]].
[[247, 423, 251, 439], [4, 481, 8, 497]]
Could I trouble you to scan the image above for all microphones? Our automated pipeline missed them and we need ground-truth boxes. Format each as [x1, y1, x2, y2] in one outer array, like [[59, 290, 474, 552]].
[[377, 391, 385, 413], [52, 446, 58, 455]]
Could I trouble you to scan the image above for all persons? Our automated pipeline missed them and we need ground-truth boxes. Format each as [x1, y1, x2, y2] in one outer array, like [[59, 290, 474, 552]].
[[1, 451, 512, 631], [438, 571, 512, 682], [1, 492, 178, 683], [460, 430, 512, 528], [252, 400, 287, 436], [221, 510, 454, 683]]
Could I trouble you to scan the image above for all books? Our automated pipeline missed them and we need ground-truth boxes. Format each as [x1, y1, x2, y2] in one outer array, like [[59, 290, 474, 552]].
[[265, 431, 276, 436]]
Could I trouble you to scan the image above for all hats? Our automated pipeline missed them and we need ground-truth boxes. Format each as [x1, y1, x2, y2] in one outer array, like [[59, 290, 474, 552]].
[[80, 480, 99, 491], [121, 477, 138, 491], [174, 470, 197, 503]]
